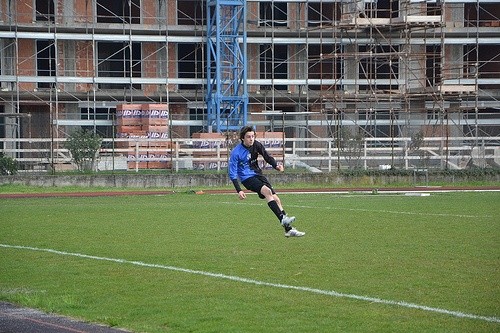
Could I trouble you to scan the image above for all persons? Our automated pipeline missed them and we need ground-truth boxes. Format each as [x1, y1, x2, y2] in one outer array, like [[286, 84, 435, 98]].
[[228, 126, 305, 238]]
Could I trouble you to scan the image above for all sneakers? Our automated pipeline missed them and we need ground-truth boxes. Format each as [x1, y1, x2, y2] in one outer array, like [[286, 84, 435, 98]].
[[280, 213, 295, 227], [285, 227, 305, 237]]
